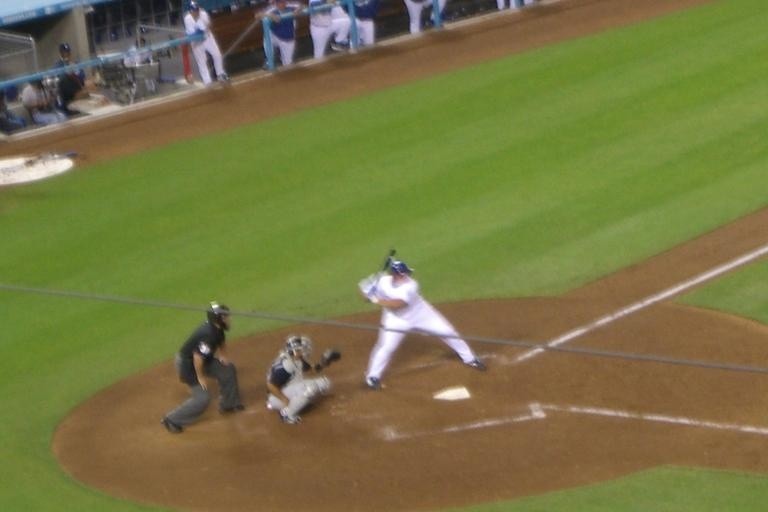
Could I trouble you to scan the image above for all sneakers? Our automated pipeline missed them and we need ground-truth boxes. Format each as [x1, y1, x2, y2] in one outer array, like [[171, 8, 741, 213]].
[[464, 357, 486, 371], [365, 376, 384, 391], [279, 407, 302, 425], [160, 417, 182, 433], [218, 402, 244, 415]]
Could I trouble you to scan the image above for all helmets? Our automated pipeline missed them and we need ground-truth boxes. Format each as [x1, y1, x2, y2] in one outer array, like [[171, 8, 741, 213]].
[[208, 304, 229, 329], [390, 261, 413, 276], [287, 335, 313, 357]]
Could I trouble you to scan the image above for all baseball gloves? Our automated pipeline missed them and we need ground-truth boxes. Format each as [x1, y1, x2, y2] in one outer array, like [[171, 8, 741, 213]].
[[319, 349, 341, 367]]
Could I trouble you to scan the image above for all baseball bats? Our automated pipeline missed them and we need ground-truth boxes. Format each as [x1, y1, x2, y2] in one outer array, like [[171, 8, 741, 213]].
[[374, 249, 395, 287]]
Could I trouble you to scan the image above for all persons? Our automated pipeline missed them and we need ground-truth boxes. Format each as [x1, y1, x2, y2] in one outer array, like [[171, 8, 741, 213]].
[[254, 1, 305, 69], [404, 1, 444, 33], [20, 80, 66, 123], [264, 334, 341, 424], [359, 257, 490, 388], [163, 301, 246, 434], [341, 2, 380, 44], [183, 1, 230, 82], [123, 38, 154, 68], [308, 1, 350, 58], [56, 42, 85, 112], [496, 1, 535, 11]]
